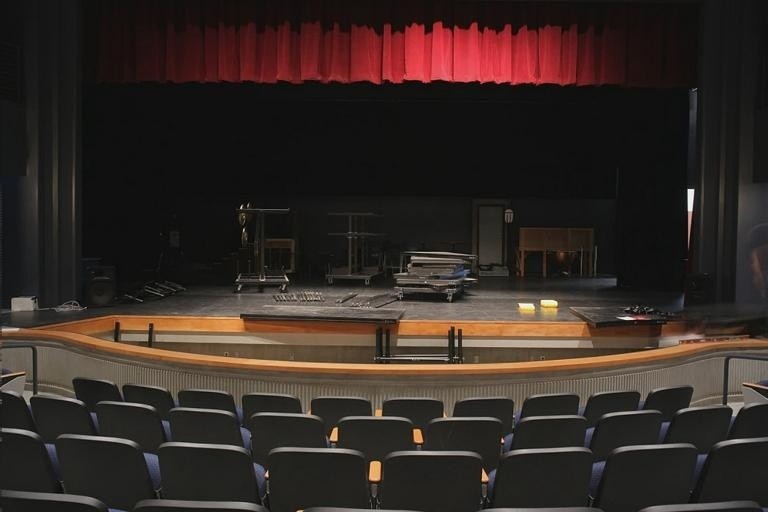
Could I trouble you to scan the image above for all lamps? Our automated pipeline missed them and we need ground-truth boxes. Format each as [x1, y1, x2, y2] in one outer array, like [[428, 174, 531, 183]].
[[503, 207, 514, 224], [516, 299, 558, 311]]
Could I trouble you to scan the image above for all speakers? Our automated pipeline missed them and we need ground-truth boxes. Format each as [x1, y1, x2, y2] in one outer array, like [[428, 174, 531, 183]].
[[80, 265, 116, 307]]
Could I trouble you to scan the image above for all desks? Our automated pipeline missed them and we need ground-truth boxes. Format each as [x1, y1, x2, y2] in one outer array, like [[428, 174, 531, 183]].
[[514, 226, 594, 278]]
